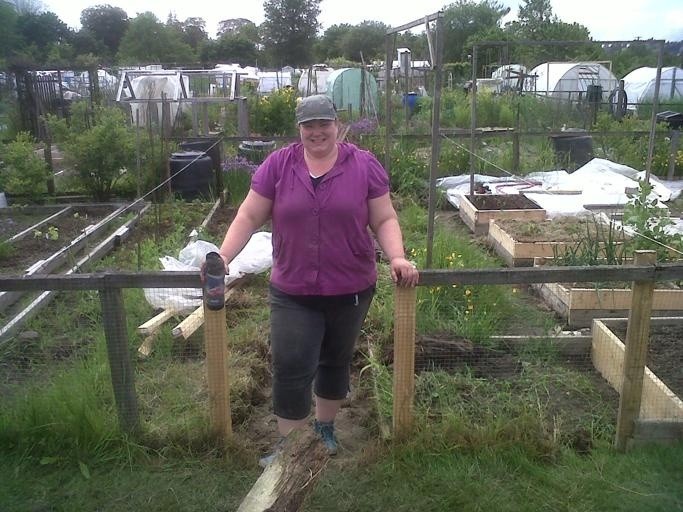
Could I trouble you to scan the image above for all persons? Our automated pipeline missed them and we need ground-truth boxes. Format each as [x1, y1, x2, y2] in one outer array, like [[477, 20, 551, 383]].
[[198, 93, 421, 470]]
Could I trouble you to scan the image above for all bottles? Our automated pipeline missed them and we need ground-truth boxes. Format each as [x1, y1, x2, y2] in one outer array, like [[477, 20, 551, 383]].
[[204, 252, 225, 310]]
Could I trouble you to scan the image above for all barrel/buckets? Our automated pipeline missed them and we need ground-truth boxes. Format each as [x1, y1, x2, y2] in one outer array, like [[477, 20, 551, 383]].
[[553, 137, 593, 173], [239, 143, 276, 163], [403, 91, 420, 115], [169, 149, 212, 201]]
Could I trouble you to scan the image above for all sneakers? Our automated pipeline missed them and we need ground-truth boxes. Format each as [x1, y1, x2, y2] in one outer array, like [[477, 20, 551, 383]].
[[258, 433, 288, 468]]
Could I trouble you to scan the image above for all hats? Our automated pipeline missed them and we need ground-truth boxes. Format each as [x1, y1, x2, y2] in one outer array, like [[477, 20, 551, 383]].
[[294, 94, 337, 125]]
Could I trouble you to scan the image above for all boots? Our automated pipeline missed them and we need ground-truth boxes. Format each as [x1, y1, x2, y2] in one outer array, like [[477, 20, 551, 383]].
[[313, 418, 338, 455]]
